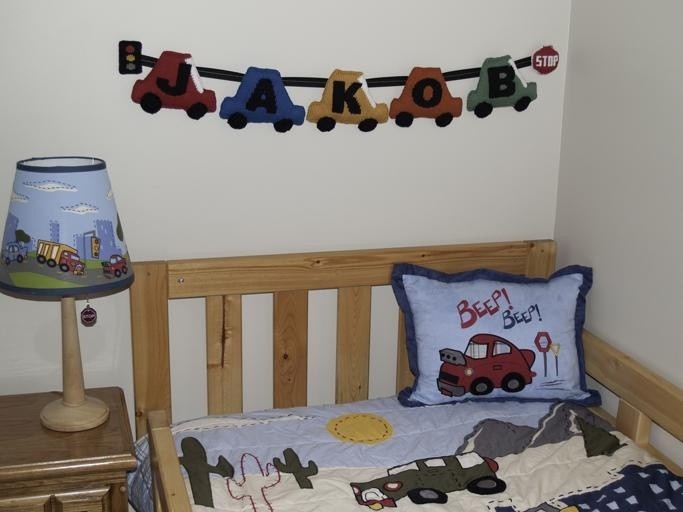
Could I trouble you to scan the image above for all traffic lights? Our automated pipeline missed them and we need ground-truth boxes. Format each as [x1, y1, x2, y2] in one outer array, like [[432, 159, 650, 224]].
[[390, 261, 603, 408]]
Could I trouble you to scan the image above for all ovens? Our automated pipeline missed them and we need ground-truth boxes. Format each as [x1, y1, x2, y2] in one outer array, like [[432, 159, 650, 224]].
[[127, 238, 683, 511]]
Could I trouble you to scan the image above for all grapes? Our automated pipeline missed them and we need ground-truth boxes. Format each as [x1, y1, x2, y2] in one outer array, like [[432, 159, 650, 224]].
[[0, 387, 137, 512]]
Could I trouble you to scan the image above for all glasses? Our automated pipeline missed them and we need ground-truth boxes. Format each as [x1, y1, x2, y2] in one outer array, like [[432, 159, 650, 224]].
[[0, 154, 135, 432]]
